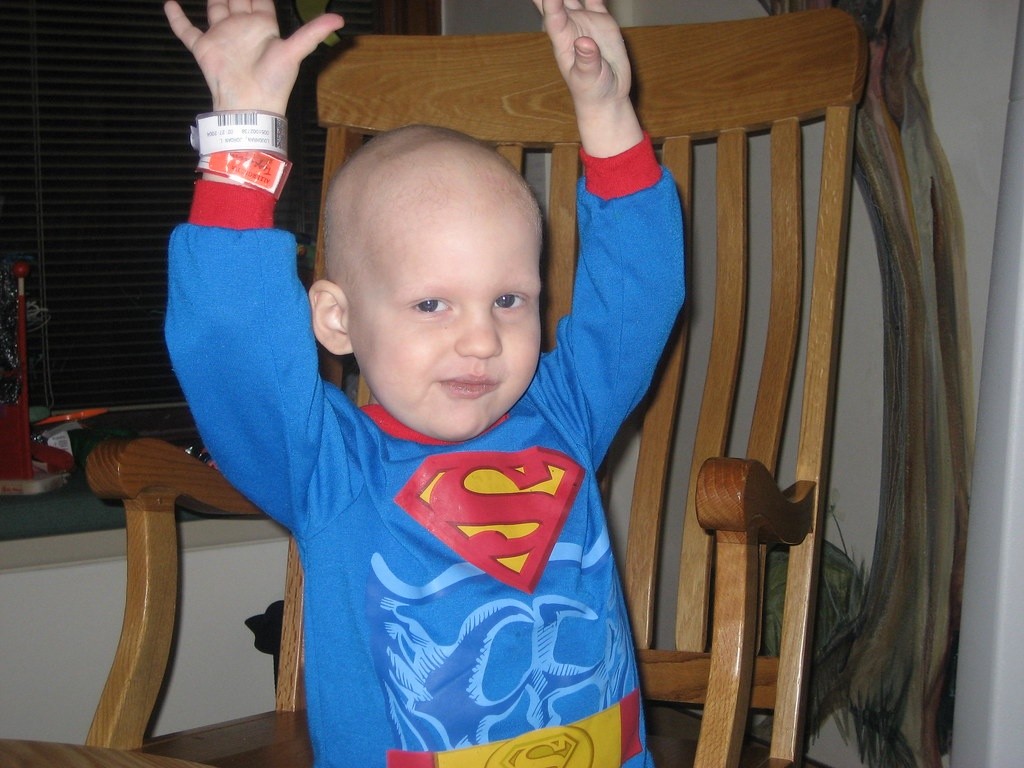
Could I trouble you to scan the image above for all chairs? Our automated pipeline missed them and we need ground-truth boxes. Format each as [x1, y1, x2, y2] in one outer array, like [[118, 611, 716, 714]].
[[0, 6, 872, 768]]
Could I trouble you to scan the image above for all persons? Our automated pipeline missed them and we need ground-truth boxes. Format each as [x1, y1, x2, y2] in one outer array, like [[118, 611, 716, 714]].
[[166, 0, 685, 768]]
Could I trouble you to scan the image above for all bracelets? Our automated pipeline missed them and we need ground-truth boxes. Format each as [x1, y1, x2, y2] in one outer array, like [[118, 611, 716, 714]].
[[191, 110, 292, 201]]
